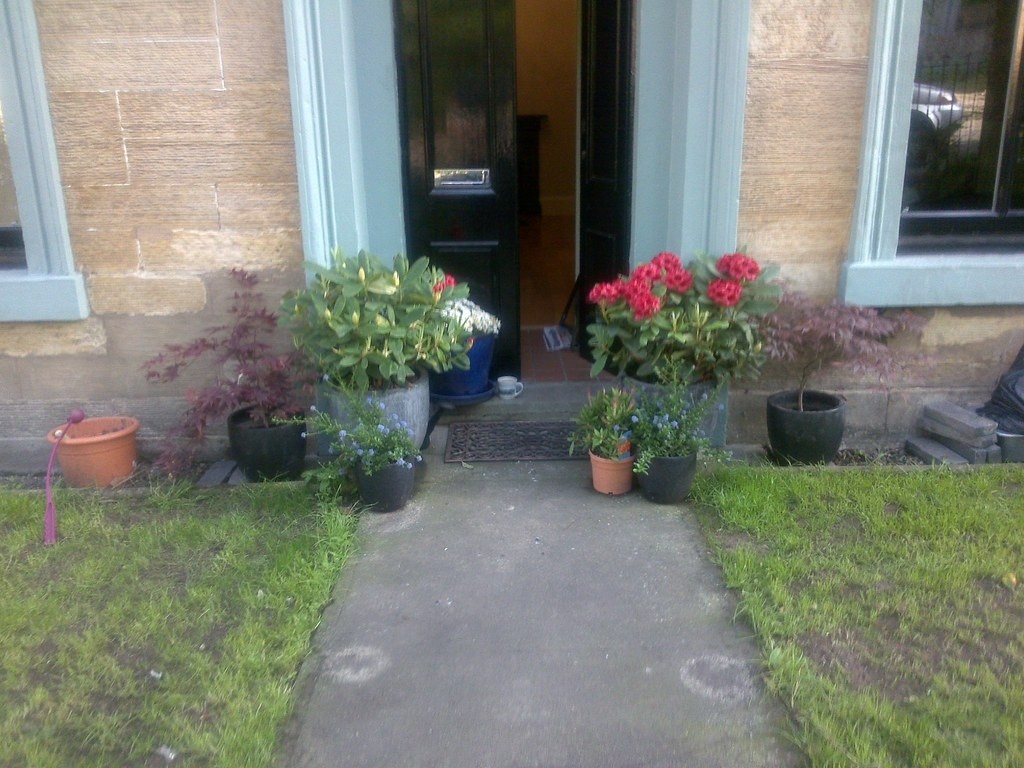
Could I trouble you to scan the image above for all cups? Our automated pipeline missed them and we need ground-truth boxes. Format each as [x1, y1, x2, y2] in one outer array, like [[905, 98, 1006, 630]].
[[497, 377, 523, 399]]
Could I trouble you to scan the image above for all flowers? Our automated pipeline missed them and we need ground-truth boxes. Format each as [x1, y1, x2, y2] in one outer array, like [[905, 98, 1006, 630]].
[[279, 249, 478, 392], [270, 368, 425, 471], [431, 273, 503, 339], [568, 243, 785, 472]]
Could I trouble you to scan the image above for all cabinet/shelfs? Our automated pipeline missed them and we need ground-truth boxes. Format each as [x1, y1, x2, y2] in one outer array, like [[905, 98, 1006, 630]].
[[516, 114, 548, 219]]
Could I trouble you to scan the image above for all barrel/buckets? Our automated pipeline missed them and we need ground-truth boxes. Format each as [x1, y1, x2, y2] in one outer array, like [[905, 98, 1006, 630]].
[[49, 416, 138, 489]]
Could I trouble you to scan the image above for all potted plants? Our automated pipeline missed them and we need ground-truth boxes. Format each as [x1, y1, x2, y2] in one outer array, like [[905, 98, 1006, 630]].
[[141, 265, 318, 481], [743, 279, 934, 462]]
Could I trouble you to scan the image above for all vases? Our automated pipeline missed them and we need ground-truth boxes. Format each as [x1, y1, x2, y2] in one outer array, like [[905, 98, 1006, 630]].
[[354, 452, 416, 513], [430, 332, 498, 405], [322, 364, 431, 461], [624, 372, 731, 446], [638, 451, 697, 503], [589, 448, 637, 495], [47, 416, 139, 487]]
